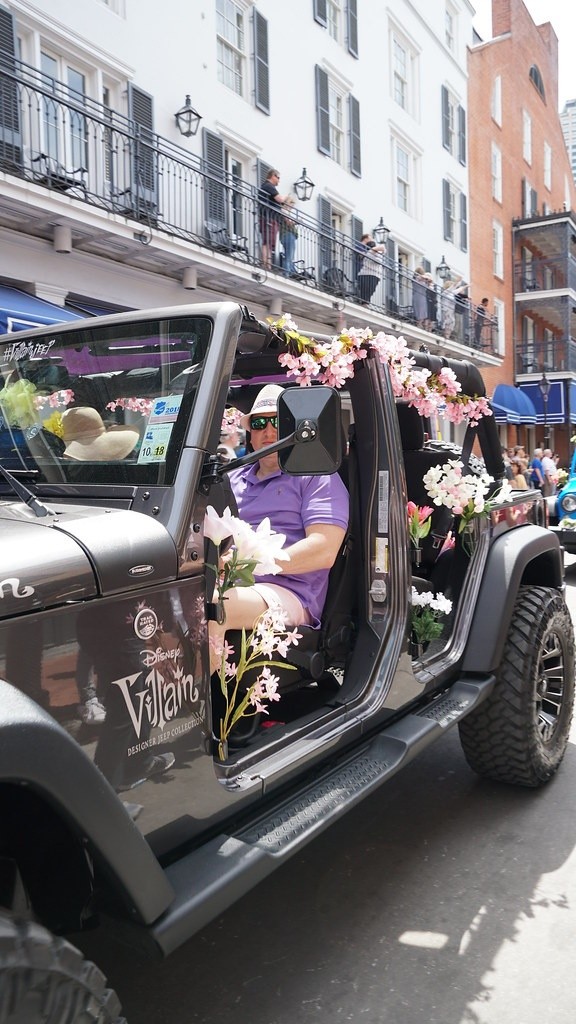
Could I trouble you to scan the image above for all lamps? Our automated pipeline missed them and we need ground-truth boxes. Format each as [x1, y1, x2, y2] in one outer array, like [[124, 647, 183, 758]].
[[293, 168, 314, 201], [174, 94, 203, 137], [53, 224, 73, 254], [436, 255, 451, 279], [182, 266, 198, 290], [268, 298, 284, 316], [372, 216, 390, 244]]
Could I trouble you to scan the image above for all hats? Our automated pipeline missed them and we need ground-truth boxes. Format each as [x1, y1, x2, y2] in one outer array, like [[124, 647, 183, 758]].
[[239, 384, 285, 432], [57, 407, 139, 460], [514, 445, 524, 451]]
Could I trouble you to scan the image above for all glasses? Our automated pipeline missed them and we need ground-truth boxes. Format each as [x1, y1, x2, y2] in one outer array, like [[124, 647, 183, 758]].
[[249, 415, 278, 430]]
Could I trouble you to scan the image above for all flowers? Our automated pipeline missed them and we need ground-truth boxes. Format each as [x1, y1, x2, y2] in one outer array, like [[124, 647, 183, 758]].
[[33, 389, 152, 416], [265, 312, 493, 428], [405, 501, 434, 567], [408, 586, 453, 646], [435, 531, 457, 563], [423, 459, 516, 534], [196, 505, 304, 763]]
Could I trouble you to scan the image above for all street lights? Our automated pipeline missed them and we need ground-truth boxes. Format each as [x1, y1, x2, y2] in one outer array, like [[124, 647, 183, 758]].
[[538, 371, 552, 449]]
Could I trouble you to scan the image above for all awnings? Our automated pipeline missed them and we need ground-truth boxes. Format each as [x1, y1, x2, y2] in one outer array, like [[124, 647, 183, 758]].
[[491, 383, 537, 426], [0, 282, 91, 353], [68, 301, 124, 318]]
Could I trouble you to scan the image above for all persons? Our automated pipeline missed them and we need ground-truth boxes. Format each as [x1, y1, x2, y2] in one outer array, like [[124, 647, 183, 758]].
[[441, 276, 471, 339], [279, 192, 299, 278], [258, 169, 292, 270], [541, 449, 560, 497], [532, 449, 545, 498], [357, 241, 386, 309], [220, 402, 247, 457], [491, 445, 534, 526], [473, 297, 488, 351], [411, 266, 432, 329], [454, 286, 472, 344], [424, 272, 438, 332], [355, 234, 371, 303], [209, 385, 350, 681]]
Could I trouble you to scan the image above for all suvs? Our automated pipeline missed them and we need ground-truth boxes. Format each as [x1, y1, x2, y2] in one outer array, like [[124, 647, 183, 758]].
[[0, 300, 576, 1024], [545, 447, 576, 554]]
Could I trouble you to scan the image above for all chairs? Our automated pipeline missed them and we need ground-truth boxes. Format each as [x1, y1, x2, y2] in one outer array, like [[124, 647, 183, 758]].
[[270, 245, 318, 288], [325, 267, 362, 303], [519, 354, 538, 373], [107, 183, 165, 228], [23, 149, 89, 202], [385, 299, 474, 346], [202, 220, 251, 263], [218, 446, 361, 743]]
